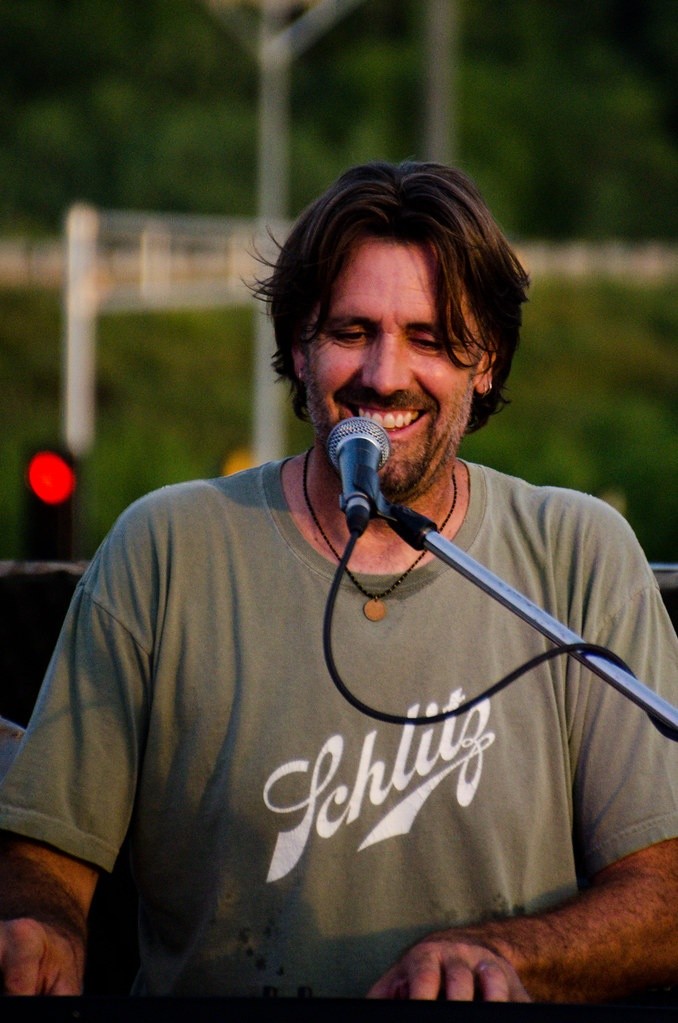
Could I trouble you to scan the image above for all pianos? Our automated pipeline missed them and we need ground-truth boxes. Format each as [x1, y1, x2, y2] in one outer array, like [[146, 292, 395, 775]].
[[1, 994, 676, 1022]]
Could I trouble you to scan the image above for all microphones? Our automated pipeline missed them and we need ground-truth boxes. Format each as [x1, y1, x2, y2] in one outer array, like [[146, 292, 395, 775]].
[[323, 417, 390, 537]]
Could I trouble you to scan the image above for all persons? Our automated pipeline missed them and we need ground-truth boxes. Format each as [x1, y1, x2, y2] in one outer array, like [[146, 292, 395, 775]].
[[0, 164, 678, 1002]]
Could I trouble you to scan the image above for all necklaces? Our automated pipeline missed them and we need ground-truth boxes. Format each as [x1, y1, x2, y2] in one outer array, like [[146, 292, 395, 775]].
[[302, 444, 457, 622]]
